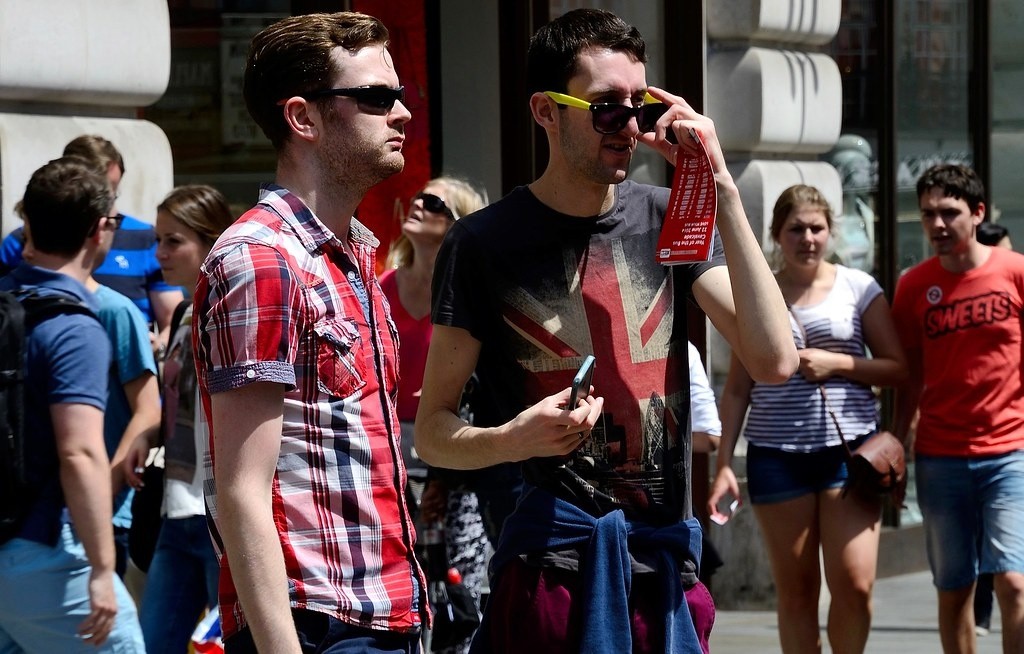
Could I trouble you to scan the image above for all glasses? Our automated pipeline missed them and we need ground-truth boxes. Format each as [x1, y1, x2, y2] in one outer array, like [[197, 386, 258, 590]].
[[415, 192, 457, 223], [543, 90, 666, 135], [275, 84, 406, 111], [104, 214, 125, 229]]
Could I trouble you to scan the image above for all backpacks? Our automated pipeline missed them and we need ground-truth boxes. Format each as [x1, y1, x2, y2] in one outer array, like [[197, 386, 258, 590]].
[[0, 291, 102, 541]]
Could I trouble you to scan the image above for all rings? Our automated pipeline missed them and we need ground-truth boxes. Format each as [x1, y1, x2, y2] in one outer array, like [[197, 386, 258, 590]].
[[579, 432, 584, 439]]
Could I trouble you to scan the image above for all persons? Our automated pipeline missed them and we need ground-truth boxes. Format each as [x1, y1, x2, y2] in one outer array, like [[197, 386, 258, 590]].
[[190, 13, 433, 654], [124, 186, 237, 654], [377, 176, 489, 470], [14, 200, 163, 583], [688, 341, 722, 454], [707, 184, 907, 654], [972, 223, 1013, 636], [0, 135, 186, 397], [414, 8, 800, 654], [889, 164, 1024, 654], [0, 157, 147, 654]]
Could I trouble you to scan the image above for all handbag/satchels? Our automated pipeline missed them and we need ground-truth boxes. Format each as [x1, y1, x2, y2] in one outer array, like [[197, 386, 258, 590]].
[[844, 431, 909, 494], [128, 460, 168, 572]]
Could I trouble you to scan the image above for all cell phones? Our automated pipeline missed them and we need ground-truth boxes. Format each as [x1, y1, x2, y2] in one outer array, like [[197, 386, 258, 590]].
[[568, 355, 596, 411]]
